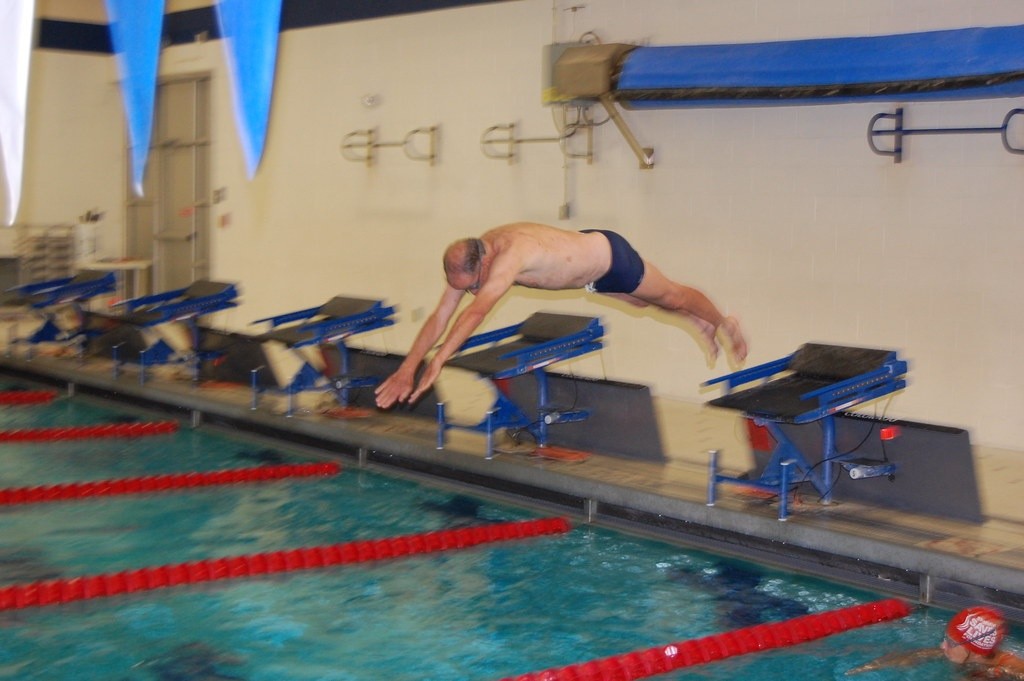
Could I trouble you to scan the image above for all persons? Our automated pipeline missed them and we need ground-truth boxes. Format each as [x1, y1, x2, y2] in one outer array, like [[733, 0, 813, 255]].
[[376, 222, 747, 408], [940, 607, 1008, 658]]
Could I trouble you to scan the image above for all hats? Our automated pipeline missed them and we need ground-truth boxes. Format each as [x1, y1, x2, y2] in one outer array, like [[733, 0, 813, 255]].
[[946, 606, 1007, 656]]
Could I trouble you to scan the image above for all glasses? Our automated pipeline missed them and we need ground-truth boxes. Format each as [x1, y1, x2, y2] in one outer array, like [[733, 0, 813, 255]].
[[464, 263, 481, 294], [943, 635, 958, 648]]
